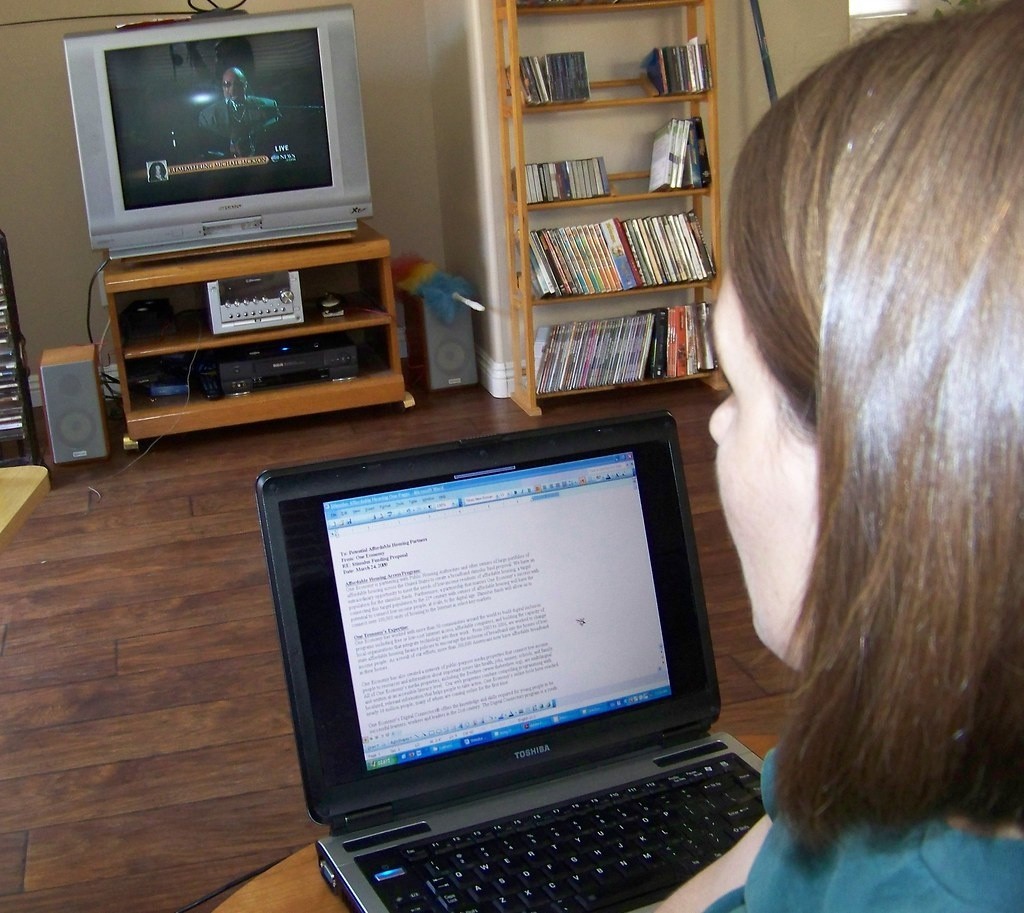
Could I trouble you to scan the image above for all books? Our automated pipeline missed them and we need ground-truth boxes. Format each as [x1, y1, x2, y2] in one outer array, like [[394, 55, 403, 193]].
[[642, 40, 713, 95], [512, 211, 716, 298], [647, 117, 712, 191], [504, 51, 591, 105], [534, 301, 715, 393], [524, 156, 610, 205]]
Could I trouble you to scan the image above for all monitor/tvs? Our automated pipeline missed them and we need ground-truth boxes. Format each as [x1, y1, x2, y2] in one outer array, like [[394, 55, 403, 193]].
[[64, 4, 372, 261]]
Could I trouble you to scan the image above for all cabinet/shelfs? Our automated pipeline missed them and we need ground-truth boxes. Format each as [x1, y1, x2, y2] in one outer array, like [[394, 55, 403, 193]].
[[493, 0, 728, 417]]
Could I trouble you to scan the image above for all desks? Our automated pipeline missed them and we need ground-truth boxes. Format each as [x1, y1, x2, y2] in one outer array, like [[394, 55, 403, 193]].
[[102, 217, 416, 452]]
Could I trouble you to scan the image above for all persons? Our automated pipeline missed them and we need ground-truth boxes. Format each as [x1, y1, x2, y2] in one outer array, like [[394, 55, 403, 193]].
[[657, 1, 1024, 913], [148, 161, 168, 183], [196, 68, 282, 144]]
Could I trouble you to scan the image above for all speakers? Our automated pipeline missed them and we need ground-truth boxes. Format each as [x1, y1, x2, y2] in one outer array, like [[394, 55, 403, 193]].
[[39, 345, 109, 463], [404, 296, 477, 392]]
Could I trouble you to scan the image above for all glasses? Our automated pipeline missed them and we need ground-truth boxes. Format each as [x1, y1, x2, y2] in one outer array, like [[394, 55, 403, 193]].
[[222, 81, 245, 87]]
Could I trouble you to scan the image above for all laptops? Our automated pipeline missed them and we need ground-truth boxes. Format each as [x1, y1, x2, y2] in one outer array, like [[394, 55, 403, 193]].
[[252, 406, 772, 913]]
